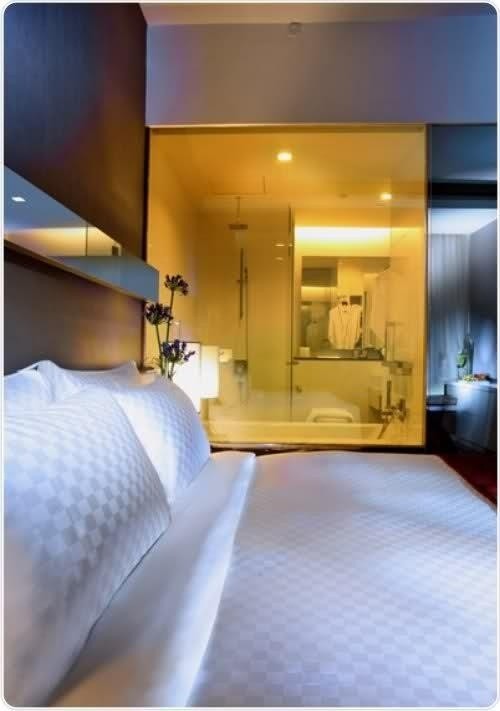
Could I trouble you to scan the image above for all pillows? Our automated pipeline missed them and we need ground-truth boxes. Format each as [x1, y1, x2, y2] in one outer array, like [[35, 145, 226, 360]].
[[64, 360, 210, 506], [2, 360, 174, 707]]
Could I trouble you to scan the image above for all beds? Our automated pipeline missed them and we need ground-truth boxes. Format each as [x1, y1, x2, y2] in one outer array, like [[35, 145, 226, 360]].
[[3, 360, 498, 709]]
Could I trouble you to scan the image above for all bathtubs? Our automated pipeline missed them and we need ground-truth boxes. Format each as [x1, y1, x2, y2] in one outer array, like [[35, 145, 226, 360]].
[[221, 423, 409, 444]]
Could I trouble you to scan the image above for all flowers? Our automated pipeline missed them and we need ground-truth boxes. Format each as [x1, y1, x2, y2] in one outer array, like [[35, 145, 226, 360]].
[[143, 273, 195, 380]]
